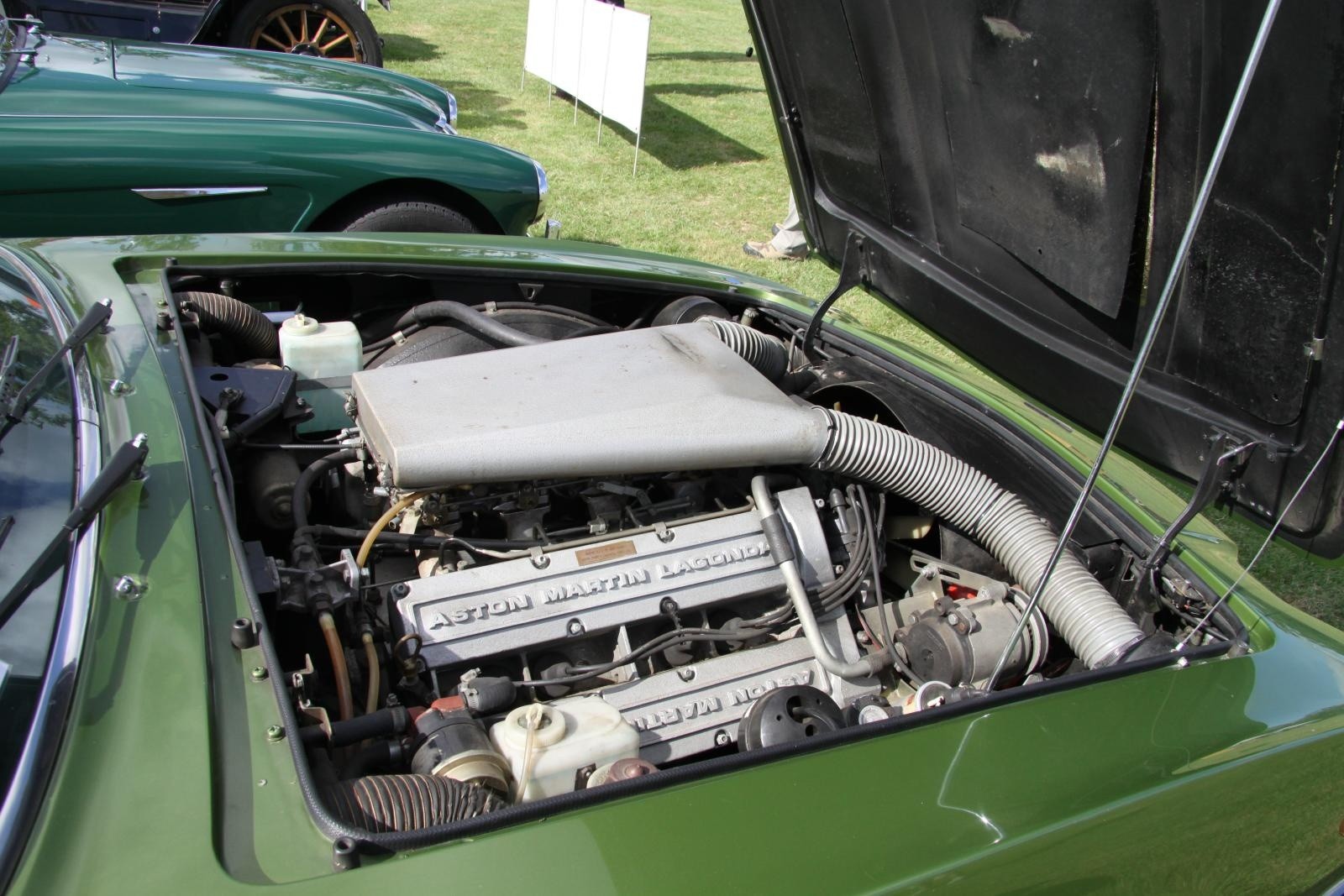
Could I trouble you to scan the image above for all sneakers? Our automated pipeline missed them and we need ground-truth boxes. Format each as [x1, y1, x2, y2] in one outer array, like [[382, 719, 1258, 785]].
[[773, 223, 782, 234], [743, 241, 807, 262]]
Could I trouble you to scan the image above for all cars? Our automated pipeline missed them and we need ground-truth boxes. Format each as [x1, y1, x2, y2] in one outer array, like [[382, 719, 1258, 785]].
[[0, 0, 392, 68], [0, 0, 1344, 896], [0, 9, 554, 240]]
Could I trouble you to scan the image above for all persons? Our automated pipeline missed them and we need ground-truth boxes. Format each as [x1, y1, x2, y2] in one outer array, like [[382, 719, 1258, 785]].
[[745, 182, 811, 260]]
